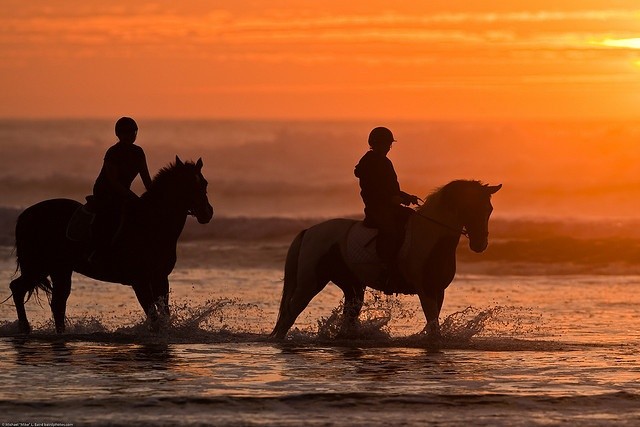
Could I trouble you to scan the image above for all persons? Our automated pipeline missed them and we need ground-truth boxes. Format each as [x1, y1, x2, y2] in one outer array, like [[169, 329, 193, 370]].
[[354, 127, 417, 295], [93, 117, 152, 285]]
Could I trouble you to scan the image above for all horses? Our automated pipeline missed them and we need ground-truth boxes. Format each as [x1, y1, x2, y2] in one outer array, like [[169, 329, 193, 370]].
[[269, 180, 502, 351], [9, 155, 213, 334]]
